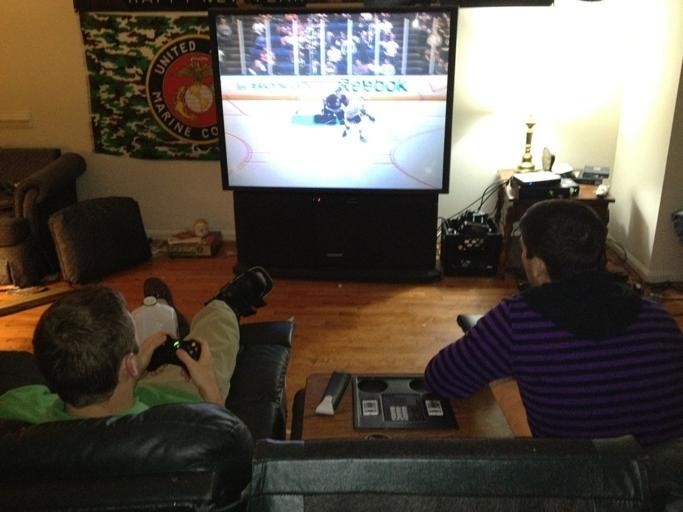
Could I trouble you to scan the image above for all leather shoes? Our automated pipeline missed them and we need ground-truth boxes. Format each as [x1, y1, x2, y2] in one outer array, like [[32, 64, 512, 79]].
[[216, 272, 267, 316], [142, 278, 185, 332]]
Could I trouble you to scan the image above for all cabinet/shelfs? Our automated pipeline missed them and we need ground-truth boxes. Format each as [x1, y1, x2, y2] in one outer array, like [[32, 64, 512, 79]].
[[495, 166, 617, 286], [233, 189, 438, 281]]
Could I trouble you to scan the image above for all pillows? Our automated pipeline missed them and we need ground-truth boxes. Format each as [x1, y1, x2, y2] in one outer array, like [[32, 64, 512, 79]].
[[45, 195, 151, 286]]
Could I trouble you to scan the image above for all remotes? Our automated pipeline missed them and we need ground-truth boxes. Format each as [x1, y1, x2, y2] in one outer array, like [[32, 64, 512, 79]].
[[312, 369, 352, 416]]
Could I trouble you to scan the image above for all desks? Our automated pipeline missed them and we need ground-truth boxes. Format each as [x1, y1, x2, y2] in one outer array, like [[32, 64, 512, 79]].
[[299, 371, 513, 439]]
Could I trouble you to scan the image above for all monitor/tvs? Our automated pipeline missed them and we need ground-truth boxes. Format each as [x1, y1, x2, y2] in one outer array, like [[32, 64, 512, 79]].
[[207, 5, 460, 195]]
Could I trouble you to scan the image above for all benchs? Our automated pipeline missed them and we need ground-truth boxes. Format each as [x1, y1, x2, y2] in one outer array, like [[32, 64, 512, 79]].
[[246, 436, 682, 512]]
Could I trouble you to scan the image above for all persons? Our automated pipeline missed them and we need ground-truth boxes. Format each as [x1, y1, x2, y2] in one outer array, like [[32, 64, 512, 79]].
[[424, 199, 682, 446], [216, 12, 449, 143], [0, 266, 272, 425]]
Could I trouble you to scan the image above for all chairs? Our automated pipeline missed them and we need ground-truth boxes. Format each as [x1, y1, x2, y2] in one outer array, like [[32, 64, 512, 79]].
[[0, 145, 85, 289], [0, 313, 296, 512]]
